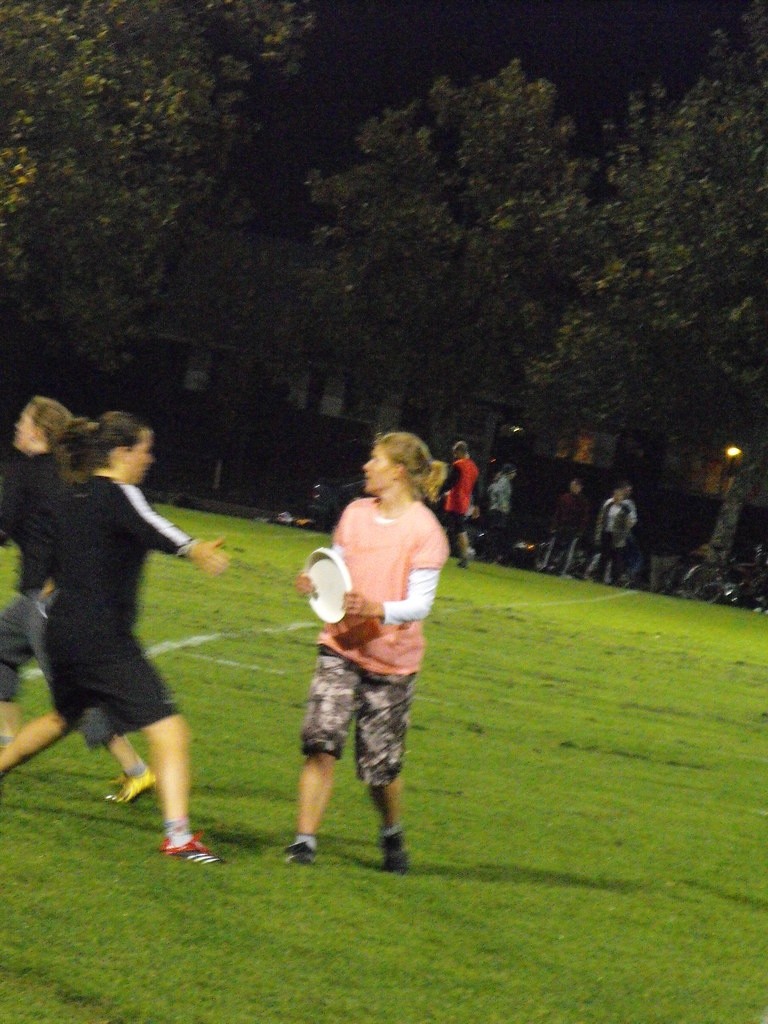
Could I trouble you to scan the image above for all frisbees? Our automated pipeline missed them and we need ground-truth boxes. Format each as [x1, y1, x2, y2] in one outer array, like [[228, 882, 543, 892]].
[[303, 548, 353, 624]]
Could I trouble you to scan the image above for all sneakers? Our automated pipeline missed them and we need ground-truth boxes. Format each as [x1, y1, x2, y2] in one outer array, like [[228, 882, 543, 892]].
[[158, 829, 224, 864], [104, 768, 157, 804]]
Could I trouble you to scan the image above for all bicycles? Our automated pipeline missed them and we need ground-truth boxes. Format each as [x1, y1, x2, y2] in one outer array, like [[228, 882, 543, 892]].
[[678, 541, 768, 611]]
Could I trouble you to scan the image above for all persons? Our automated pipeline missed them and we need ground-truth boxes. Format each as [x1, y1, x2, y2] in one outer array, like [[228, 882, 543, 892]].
[[0, 413, 234, 862], [279, 432, 450, 871], [441, 440, 638, 584], [0, 397, 157, 805]]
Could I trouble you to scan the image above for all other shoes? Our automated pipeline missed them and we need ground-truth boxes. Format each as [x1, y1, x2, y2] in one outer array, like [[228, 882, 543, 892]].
[[381, 829, 410, 876], [282, 840, 320, 866], [456, 548, 476, 567]]
